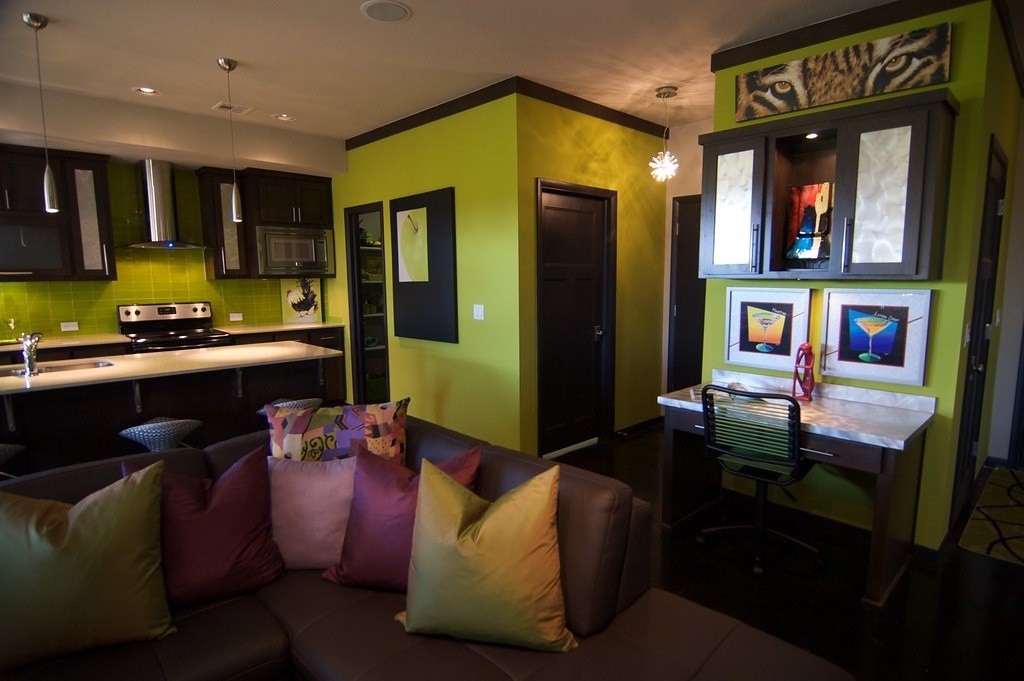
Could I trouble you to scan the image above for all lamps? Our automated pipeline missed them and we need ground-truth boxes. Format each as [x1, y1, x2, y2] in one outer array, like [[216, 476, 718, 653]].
[[648, 86, 678, 181], [22, 10, 61, 213], [218, 58, 246, 222]]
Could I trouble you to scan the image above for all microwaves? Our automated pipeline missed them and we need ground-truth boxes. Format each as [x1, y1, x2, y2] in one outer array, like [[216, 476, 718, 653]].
[[266, 234, 328, 271]]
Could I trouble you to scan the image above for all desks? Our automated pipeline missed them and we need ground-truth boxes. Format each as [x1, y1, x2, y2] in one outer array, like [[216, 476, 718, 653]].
[[0, 337, 343, 457], [657, 372, 938, 619]]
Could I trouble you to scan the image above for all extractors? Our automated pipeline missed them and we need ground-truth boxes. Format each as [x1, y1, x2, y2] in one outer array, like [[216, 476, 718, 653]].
[[115, 158, 208, 250]]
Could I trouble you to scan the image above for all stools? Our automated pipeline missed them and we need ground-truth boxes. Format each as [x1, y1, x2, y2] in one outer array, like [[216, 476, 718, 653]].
[[119, 417, 202, 454]]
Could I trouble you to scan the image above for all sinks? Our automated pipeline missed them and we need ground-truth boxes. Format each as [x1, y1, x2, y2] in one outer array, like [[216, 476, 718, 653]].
[[0, 367, 27, 377], [35, 361, 114, 373]]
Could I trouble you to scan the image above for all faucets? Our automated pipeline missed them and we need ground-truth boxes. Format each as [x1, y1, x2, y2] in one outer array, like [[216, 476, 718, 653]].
[[21, 331, 43, 375]]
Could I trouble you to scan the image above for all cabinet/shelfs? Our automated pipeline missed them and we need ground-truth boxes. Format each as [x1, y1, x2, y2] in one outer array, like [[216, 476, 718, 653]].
[[194, 166, 336, 280], [0, 143, 117, 283], [357, 245, 388, 401], [695, 86, 960, 282], [1, 327, 346, 478]]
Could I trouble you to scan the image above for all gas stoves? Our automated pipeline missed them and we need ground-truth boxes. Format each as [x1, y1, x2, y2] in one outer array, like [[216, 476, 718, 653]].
[[117, 301, 230, 345]]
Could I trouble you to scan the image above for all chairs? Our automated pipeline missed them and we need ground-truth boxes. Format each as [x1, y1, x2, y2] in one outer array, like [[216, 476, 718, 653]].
[[686, 386, 826, 590]]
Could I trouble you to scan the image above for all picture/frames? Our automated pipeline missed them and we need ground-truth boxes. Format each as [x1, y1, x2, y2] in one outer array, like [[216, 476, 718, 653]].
[[820, 288, 933, 388], [279, 276, 326, 324], [724, 286, 812, 373]]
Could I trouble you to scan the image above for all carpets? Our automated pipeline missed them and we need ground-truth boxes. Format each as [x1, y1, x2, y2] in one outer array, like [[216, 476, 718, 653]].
[[640, 481, 1024, 681], [958, 465, 1023, 565]]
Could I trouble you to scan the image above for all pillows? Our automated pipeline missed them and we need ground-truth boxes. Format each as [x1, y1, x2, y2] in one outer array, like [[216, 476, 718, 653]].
[[0, 459, 177, 652], [395, 458, 580, 654], [321, 445, 485, 594], [265, 456, 362, 571], [158, 447, 286, 610], [257, 396, 412, 469]]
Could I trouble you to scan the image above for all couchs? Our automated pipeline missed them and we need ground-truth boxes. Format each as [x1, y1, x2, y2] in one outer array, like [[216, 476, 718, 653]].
[[0, 402, 650, 681]]
